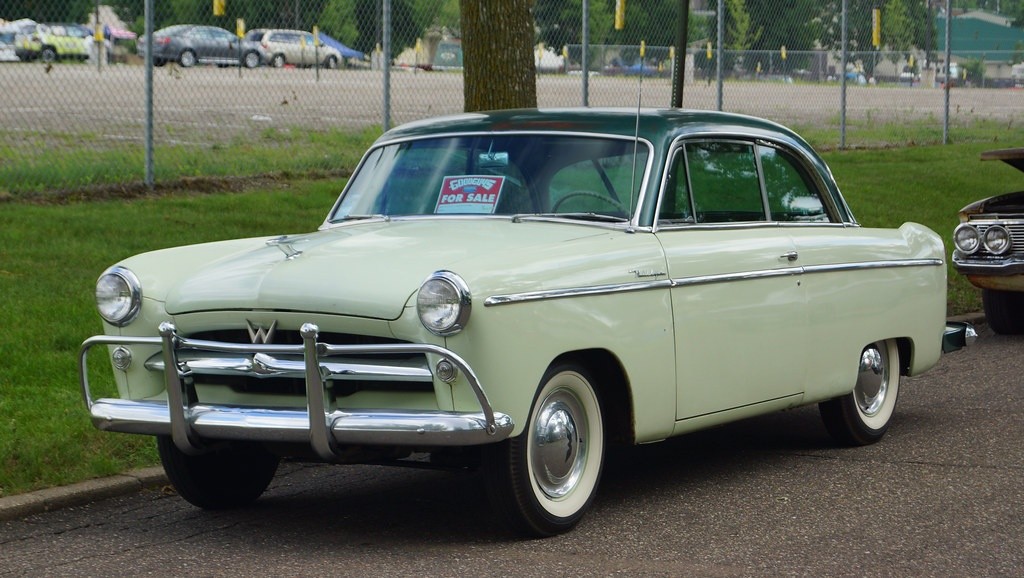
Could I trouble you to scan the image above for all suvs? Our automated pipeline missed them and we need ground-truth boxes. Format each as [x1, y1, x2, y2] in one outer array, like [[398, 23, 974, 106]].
[[244, 28, 342, 69], [14, 22, 111, 64]]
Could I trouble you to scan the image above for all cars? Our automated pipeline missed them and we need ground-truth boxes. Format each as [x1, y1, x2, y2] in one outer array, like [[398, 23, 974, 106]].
[[952, 151, 1024, 333], [136, 24, 263, 68], [77, 105, 976, 536], [604, 57, 658, 76], [0, 40, 20, 62]]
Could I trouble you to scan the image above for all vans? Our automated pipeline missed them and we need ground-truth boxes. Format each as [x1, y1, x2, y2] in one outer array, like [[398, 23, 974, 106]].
[[937, 63, 958, 82], [1012, 64, 1024, 86], [900, 64, 922, 82]]
